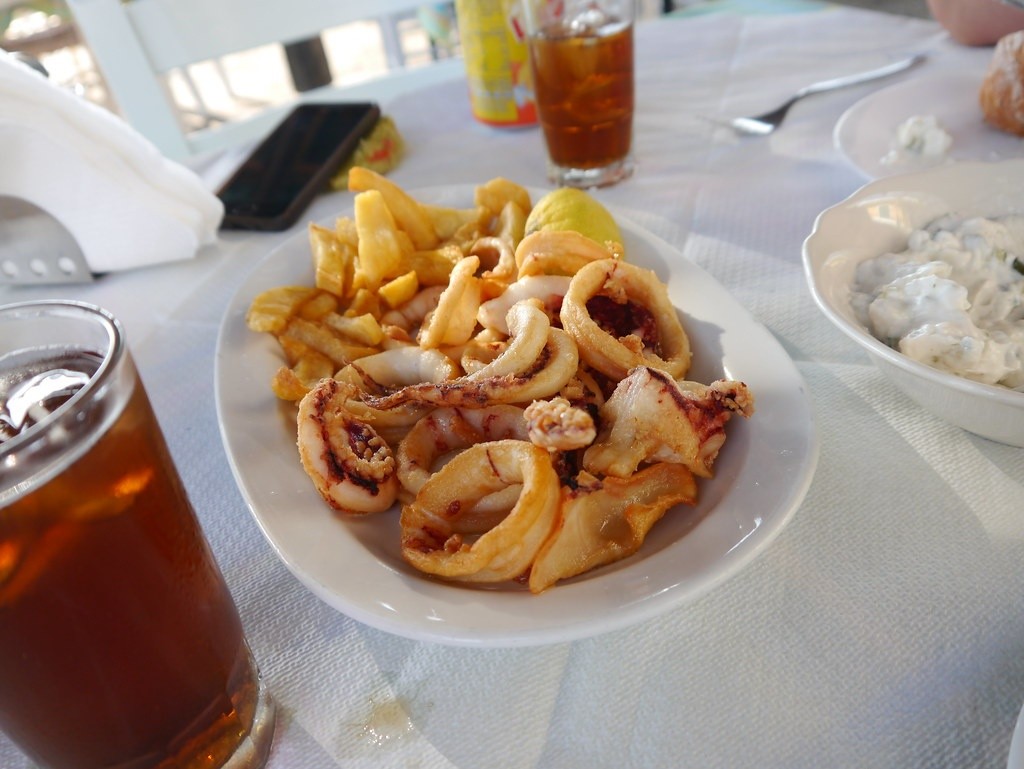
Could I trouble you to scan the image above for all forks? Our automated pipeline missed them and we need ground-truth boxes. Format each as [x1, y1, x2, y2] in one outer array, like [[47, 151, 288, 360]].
[[706, 28, 950, 140]]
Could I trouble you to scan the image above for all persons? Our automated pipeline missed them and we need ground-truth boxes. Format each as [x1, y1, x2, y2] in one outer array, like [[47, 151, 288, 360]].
[[927, 0, 1024, 48]]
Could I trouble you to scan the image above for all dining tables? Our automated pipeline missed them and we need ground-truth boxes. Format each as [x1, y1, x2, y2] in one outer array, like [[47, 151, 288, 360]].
[[1, 0, 1024, 769]]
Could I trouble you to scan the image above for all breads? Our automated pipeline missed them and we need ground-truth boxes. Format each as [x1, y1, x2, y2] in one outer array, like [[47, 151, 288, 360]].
[[979, 30, 1024, 133]]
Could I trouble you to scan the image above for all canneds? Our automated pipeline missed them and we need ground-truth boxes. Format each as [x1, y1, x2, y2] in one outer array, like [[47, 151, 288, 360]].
[[454, 0, 564, 131]]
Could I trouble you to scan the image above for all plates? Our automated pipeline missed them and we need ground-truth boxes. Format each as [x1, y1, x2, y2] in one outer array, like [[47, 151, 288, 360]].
[[833, 73, 1024, 184], [208, 201, 822, 650]]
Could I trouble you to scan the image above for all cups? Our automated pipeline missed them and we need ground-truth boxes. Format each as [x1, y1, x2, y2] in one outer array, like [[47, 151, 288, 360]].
[[521, 1, 639, 193], [0, 299, 277, 768]]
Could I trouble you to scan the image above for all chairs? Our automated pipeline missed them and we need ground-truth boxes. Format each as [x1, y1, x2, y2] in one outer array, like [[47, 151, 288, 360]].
[[65, 0, 456, 157]]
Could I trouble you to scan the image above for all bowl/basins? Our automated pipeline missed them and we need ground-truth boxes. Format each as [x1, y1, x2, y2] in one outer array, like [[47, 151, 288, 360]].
[[801, 158, 1024, 448]]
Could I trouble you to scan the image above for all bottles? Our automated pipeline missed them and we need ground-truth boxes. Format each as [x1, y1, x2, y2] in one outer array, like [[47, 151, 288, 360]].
[[454, 1, 561, 132]]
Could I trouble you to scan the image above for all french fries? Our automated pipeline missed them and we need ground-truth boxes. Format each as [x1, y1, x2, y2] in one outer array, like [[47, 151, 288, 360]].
[[242, 166, 532, 405]]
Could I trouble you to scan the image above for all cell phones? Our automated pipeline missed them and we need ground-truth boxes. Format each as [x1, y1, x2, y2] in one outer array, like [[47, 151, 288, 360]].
[[214, 102, 379, 230]]
